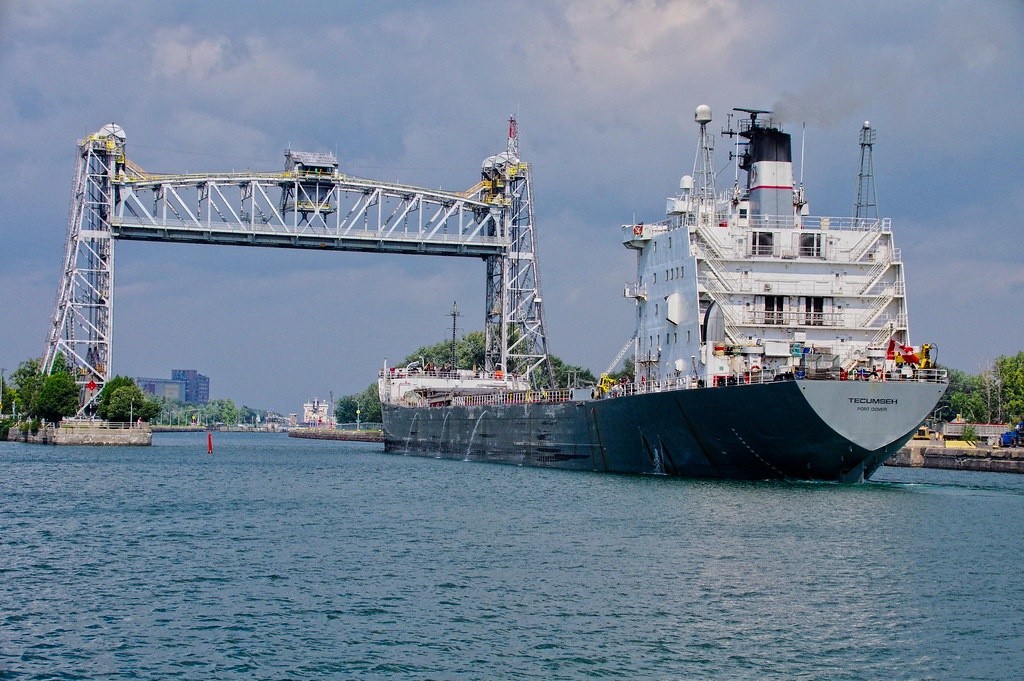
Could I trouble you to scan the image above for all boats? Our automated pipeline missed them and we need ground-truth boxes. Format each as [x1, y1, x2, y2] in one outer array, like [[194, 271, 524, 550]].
[[376, 105, 954, 485]]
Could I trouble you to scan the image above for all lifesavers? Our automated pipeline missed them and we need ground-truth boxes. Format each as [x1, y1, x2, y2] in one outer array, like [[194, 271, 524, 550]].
[[634, 226, 642, 237], [752, 364, 760, 374]]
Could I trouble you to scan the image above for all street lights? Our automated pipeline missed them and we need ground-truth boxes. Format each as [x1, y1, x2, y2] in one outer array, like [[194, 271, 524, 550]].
[[129, 397, 135, 430], [351, 399, 360, 431], [934, 406, 950, 423]]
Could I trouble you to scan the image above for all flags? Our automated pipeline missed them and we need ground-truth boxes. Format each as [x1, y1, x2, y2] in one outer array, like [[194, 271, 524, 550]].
[[885, 339, 919, 364]]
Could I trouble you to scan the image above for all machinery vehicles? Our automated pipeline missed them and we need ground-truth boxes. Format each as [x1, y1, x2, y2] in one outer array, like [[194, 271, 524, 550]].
[[997, 421, 1024, 449], [895, 343, 941, 381], [595, 335, 634, 398], [913, 425, 931, 440]]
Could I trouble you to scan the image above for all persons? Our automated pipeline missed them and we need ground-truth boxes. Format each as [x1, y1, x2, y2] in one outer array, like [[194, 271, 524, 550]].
[[800, 354, 805, 366], [609, 383, 621, 397], [591, 389, 594, 399], [596, 388, 601, 400], [138, 417, 143, 429]]
